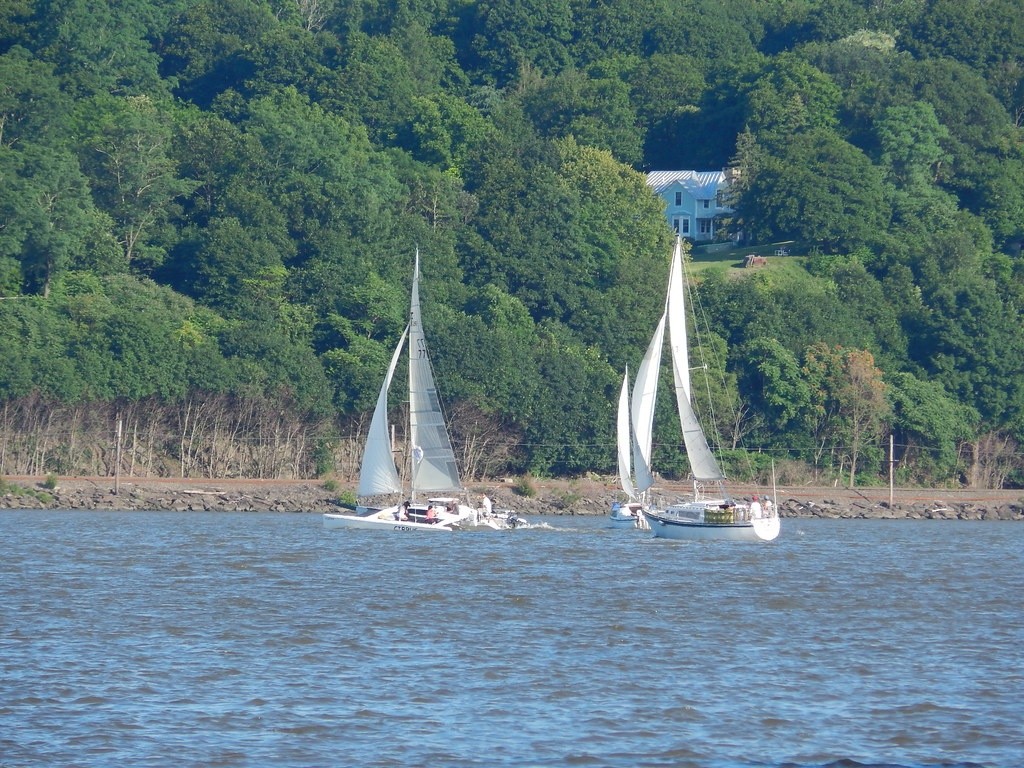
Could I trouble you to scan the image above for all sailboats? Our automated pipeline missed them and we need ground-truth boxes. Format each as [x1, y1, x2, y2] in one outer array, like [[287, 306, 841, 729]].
[[321, 242, 529, 531], [629, 234, 781, 543]]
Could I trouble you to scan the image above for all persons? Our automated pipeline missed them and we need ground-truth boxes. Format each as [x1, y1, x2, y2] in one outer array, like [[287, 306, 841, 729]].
[[425, 505, 438, 525], [480, 491, 492, 517], [396, 501, 411, 521], [749, 496, 761, 519], [762, 496, 772, 518]]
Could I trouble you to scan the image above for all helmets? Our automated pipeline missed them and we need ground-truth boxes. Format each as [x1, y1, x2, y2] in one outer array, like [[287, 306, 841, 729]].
[[752, 496, 758, 501], [764, 495, 769, 500]]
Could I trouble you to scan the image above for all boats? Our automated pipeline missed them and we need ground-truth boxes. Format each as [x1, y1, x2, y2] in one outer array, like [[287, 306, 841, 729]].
[[608, 360, 638, 529]]
